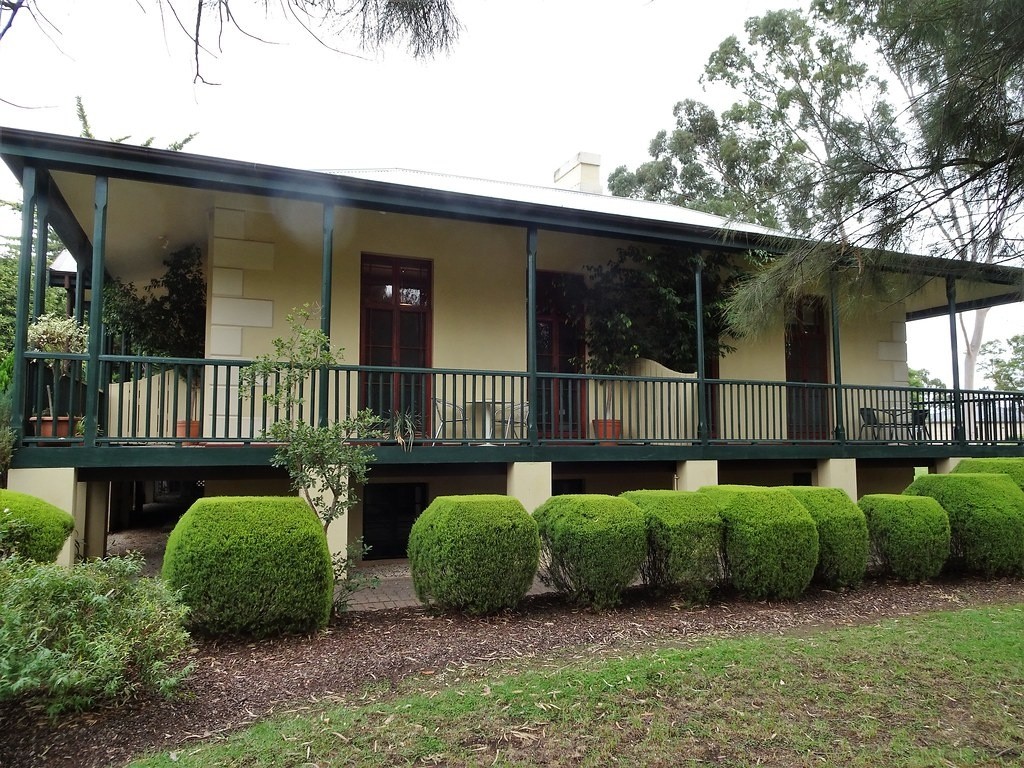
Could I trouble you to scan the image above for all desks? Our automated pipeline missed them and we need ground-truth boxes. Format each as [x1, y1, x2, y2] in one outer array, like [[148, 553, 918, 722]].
[[466, 400, 511, 446], [879, 409, 909, 440]]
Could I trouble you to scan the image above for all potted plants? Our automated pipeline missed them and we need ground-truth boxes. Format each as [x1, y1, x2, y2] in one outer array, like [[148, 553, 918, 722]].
[[27, 312, 90, 447], [553, 258, 641, 447], [101, 245, 207, 445]]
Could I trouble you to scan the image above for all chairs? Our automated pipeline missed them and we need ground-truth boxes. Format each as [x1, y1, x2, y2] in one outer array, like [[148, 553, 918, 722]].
[[909, 410, 930, 440], [490, 401, 529, 446], [858, 409, 886, 440], [430, 398, 471, 447]]
